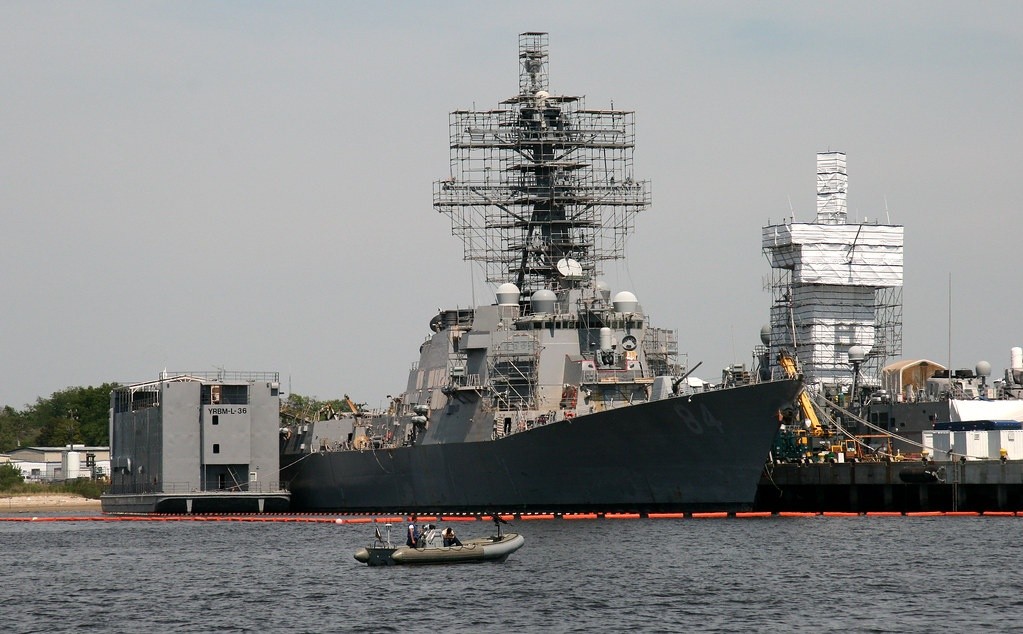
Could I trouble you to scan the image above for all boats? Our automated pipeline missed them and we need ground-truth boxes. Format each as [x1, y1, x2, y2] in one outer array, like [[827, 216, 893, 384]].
[[354, 531, 525, 568], [411, 415, 427, 424], [413, 405, 429, 413]]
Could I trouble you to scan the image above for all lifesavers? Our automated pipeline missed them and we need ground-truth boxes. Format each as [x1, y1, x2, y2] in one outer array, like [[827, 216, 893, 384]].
[[566, 412, 574, 419], [353, 436, 369, 450]]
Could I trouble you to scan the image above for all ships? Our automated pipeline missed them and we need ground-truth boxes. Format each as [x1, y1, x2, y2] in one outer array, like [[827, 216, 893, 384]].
[[159, 249, 1023, 518]]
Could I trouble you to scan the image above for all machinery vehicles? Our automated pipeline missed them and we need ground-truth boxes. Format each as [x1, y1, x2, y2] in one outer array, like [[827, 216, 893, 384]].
[[776, 347, 895, 464]]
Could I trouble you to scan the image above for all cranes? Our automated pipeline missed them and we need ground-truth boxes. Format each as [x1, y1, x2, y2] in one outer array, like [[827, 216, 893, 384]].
[[342, 392, 368, 451]]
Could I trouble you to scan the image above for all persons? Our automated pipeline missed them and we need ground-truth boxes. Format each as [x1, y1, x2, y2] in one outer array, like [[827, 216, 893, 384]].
[[918, 389, 946, 402], [279, 425, 291, 440], [406, 515, 463, 548], [497, 319, 504, 332], [517, 419, 526, 432], [979, 393, 987, 400], [100, 474, 109, 495]]
